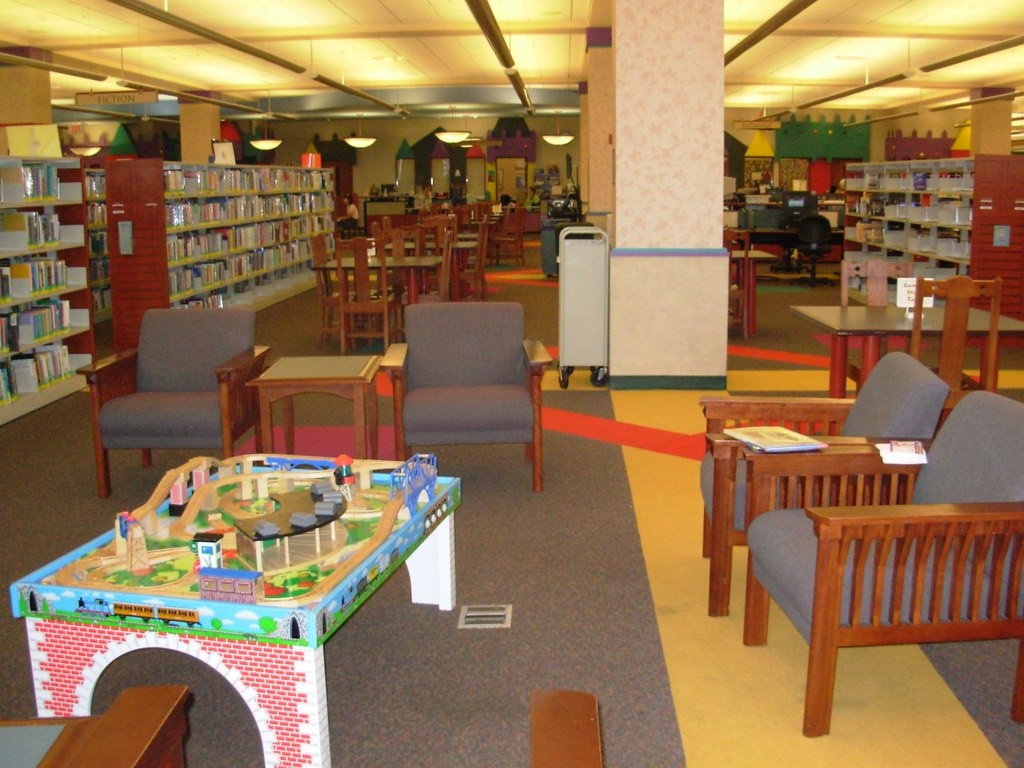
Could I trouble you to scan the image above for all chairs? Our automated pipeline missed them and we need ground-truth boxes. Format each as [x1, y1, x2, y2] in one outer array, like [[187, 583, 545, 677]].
[[76, 202, 1024, 768]]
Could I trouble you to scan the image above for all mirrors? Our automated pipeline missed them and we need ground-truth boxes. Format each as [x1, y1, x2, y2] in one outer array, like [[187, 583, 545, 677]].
[[495, 157, 524, 204]]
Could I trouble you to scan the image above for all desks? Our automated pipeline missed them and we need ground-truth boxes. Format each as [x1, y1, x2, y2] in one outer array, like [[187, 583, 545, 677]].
[[384, 242, 478, 298], [789, 307, 1024, 398], [245, 355, 384, 458], [728, 227, 842, 280], [10, 454, 461, 767], [0, 716, 102, 768], [407, 234, 478, 238], [311, 257, 445, 343], [732, 251, 777, 334]]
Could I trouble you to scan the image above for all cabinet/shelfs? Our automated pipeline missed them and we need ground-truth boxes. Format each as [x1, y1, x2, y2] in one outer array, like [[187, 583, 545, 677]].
[[0, 158, 338, 427], [844, 154, 1024, 347]]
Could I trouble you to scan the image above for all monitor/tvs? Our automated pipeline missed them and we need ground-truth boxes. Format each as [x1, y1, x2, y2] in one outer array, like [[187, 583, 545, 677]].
[[380, 184, 397, 193], [782, 191, 813, 229]]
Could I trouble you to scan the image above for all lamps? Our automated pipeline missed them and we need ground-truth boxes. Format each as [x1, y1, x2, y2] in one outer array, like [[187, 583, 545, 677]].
[[435, 107, 471, 143], [250, 121, 282, 150], [542, 113, 575, 145], [344, 115, 376, 148]]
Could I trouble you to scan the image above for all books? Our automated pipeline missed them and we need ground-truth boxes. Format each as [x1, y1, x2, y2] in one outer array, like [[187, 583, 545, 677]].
[[855, 173, 931, 244], [84, 171, 112, 314], [875, 441, 928, 465], [164, 163, 334, 308], [723, 426, 828, 453], [0, 157, 71, 405]]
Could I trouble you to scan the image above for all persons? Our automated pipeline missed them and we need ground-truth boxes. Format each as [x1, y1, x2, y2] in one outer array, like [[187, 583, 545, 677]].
[[524, 186, 539, 211], [755, 170, 773, 194], [337, 195, 359, 238]]
